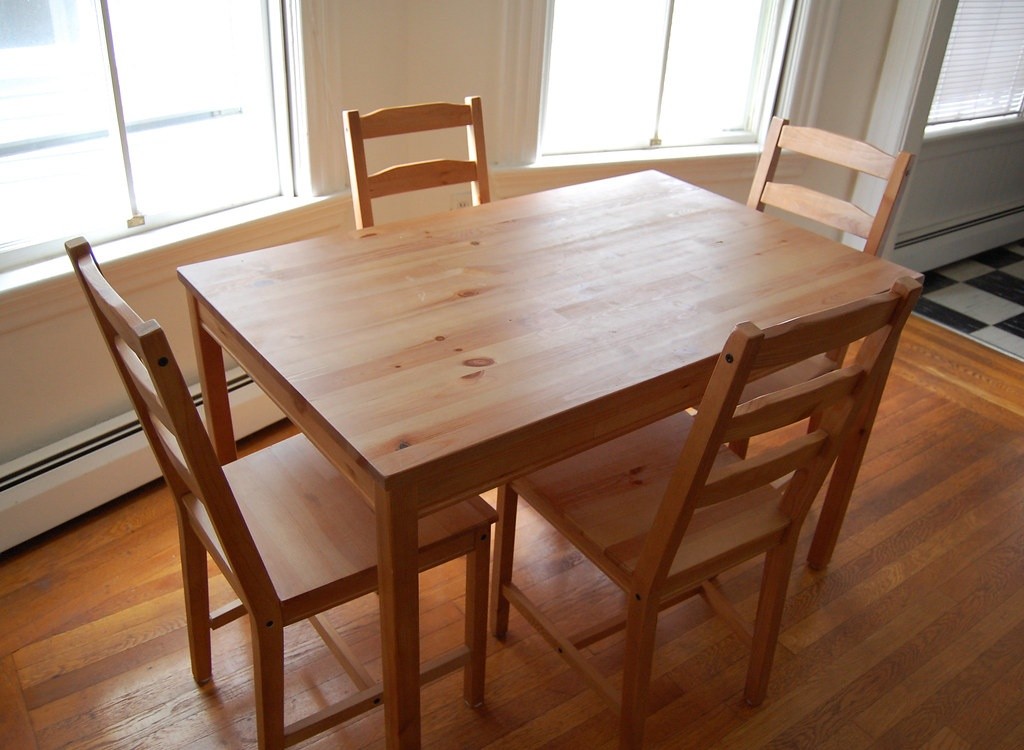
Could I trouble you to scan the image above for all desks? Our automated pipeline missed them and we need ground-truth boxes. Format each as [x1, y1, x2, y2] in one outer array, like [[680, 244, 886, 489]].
[[176, 169, 924, 750]]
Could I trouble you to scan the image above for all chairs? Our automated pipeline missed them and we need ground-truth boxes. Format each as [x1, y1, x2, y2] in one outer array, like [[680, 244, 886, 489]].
[[488, 277, 922, 750], [64, 234, 498, 748], [703, 115, 913, 462], [340, 94, 491, 234]]
